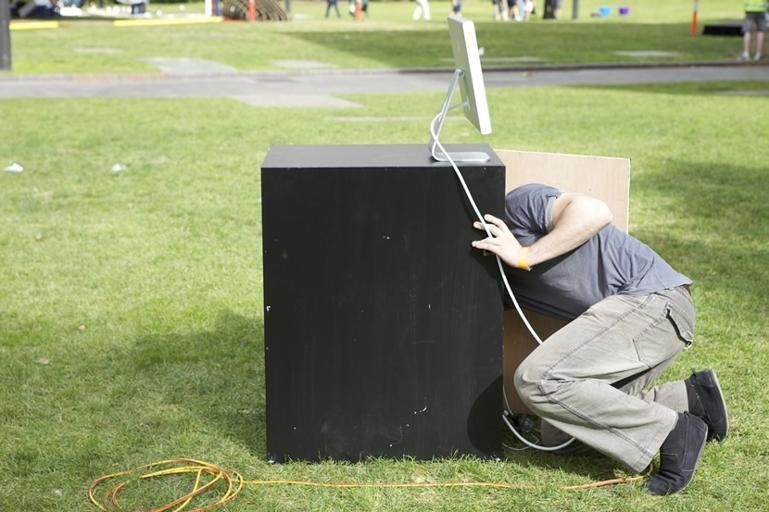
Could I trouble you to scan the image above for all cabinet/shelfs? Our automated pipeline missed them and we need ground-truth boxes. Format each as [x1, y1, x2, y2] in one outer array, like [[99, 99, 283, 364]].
[[259, 143, 634, 464]]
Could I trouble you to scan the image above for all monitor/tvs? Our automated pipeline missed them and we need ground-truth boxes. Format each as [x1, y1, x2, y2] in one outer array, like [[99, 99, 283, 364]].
[[425, 16, 493, 162]]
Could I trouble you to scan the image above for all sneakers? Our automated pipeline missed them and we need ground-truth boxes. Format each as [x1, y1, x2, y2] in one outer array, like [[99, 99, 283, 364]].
[[691, 370, 729, 442], [642, 412, 708, 495]]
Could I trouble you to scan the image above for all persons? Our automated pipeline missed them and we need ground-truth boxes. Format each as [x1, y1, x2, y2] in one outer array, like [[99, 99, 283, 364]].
[[737, 0, 769, 60], [132, 0, 146, 14], [409, 0, 558, 21], [471, 184, 729, 496], [323, 0, 342, 17]]
